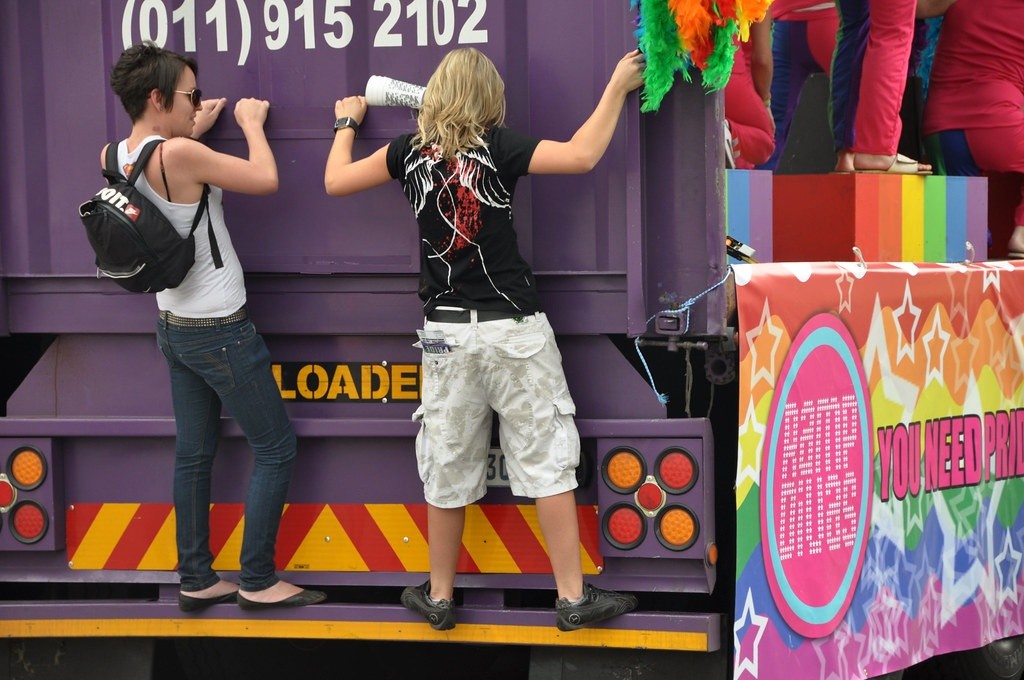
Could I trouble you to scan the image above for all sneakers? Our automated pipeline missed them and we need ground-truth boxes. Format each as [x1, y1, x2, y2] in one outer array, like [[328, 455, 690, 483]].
[[555, 583, 639, 631], [402, 586, 457, 630]]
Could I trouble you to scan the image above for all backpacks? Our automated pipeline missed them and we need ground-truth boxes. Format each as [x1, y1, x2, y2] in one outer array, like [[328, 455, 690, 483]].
[[79, 140, 224, 294]]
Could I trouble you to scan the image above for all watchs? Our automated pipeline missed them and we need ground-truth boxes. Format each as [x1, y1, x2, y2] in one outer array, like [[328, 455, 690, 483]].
[[334, 117, 359, 139]]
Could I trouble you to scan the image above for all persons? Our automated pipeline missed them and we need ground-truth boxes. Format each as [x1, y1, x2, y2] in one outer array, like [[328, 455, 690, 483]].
[[324, 47, 646, 631], [724, 0, 1024, 258], [77, 40, 328, 612]]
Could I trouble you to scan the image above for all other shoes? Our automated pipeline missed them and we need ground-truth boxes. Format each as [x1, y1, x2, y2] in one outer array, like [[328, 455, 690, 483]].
[[237, 589, 328, 610], [178, 591, 238, 612]]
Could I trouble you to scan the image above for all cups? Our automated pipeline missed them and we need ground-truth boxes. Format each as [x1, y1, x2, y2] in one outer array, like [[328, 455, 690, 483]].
[[364, 75, 426, 108]]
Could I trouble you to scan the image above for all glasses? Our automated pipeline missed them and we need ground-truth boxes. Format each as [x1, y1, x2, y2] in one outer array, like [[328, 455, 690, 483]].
[[147, 89, 202, 108]]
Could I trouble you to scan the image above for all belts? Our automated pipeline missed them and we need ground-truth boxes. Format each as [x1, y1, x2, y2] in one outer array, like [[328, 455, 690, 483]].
[[425, 308, 532, 324], [160, 308, 246, 328]]
